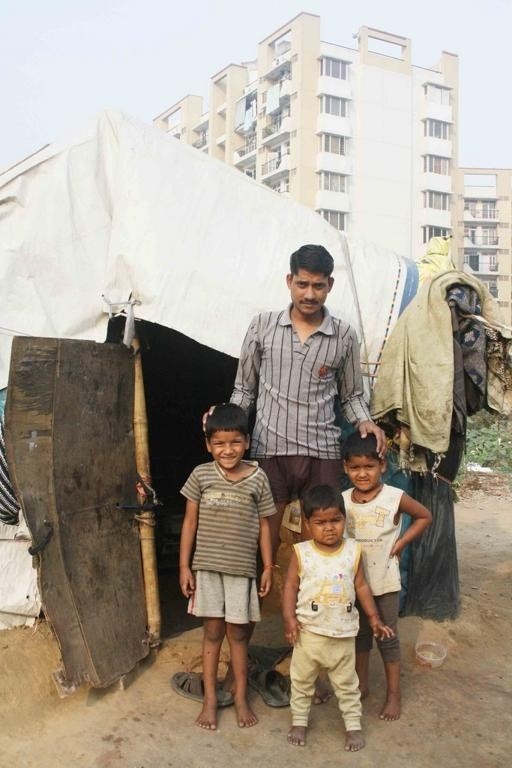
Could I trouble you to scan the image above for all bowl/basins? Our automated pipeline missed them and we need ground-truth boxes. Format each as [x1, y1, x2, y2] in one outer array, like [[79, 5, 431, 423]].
[[413, 639, 447, 668]]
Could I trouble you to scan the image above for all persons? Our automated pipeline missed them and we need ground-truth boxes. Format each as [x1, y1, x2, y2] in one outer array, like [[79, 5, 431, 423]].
[[203, 245, 389, 703], [179, 401, 277, 729], [339, 431, 432, 720], [279, 484, 395, 751]]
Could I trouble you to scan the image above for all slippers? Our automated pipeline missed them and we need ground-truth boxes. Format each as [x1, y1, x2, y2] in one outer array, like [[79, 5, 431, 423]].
[[171, 672, 234, 707], [248, 669, 291, 707]]
